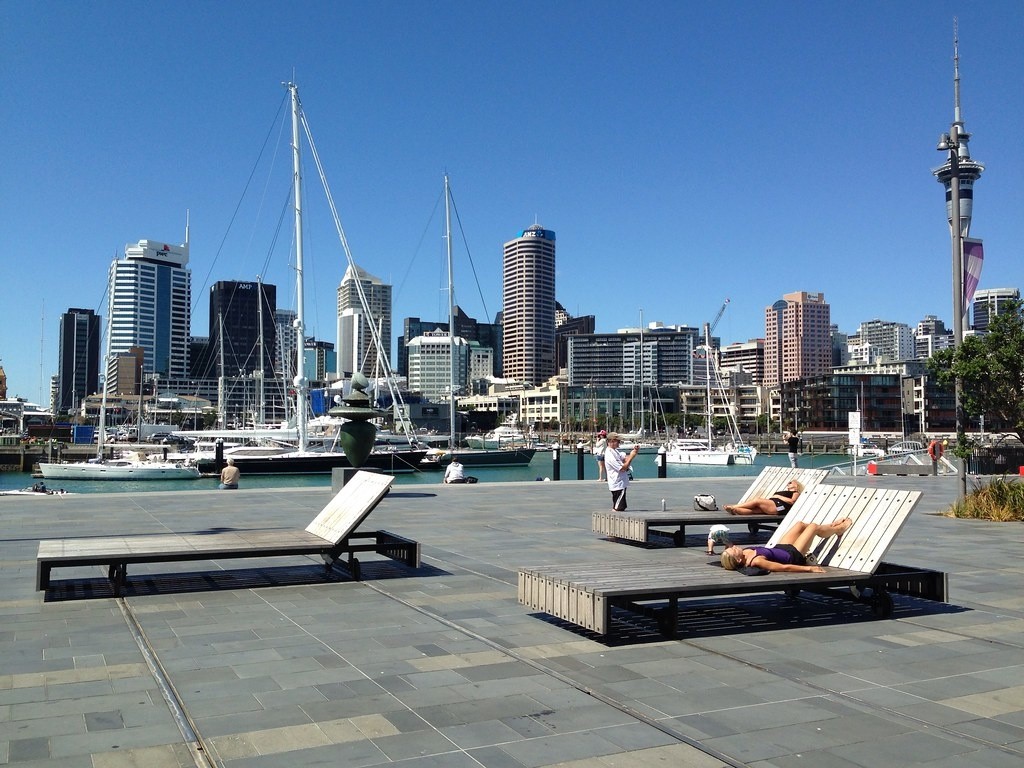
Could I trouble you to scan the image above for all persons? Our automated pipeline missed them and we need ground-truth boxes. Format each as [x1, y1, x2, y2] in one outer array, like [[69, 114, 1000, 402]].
[[444, 456, 465, 484], [604, 432, 640, 511], [32, 483, 40, 492], [720, 518, 854, 573], [722, 479, 805, 515], [218, 457, 240, 489], [706, 524, 734, 556], [595, 430, 609, 481], [110, 437, 116, 444], [39, 482, 46, 492], [782, 429, 800, 468]]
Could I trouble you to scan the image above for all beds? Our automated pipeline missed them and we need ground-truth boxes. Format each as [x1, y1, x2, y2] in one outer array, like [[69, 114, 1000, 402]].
[[590, 465, 829, 543], [518, 484, 950, 637], [35, 470, 421, 596]]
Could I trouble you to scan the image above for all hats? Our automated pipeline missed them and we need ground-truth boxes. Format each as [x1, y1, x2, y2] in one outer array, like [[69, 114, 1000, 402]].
[[607, 432, 624, 440], [597, 430, 606, 435]]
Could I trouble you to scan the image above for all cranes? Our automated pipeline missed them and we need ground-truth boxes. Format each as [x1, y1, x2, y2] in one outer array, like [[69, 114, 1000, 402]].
[[708, 297, 730, 337]]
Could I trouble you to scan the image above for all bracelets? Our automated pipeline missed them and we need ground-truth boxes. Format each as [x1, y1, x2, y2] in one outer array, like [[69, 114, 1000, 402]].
[[783, 439, 785, 440]]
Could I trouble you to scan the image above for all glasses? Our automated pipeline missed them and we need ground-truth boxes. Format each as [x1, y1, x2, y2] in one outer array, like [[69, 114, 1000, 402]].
[[789, 482, 792, 484]]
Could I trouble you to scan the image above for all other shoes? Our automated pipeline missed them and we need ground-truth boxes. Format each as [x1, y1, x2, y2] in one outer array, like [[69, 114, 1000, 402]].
[[706, 550, 713, 555], [597, 479, 602, 482]]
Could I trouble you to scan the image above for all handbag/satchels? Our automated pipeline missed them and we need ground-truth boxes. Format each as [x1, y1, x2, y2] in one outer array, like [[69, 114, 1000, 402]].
[[693, 494, 718, 511]]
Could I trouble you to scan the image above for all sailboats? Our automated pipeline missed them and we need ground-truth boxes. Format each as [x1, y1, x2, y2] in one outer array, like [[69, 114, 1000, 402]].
[[39, 67, 760, 482]]
